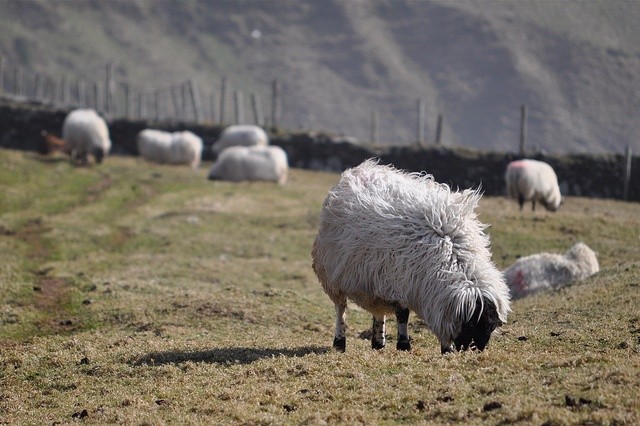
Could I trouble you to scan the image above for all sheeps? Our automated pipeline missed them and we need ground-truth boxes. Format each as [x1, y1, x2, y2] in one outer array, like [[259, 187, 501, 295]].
[[206, 144, 288, 185], [310, 157, 513, 354], [499, 242, 600, 301], [211, 123, 268, 161], [505, 158, 564, 214], [62, 107, 112, 165], [136, 126, 203, 167]]
